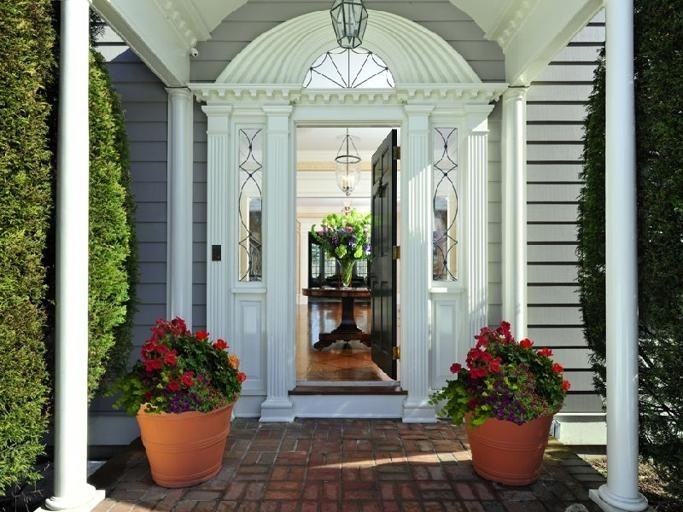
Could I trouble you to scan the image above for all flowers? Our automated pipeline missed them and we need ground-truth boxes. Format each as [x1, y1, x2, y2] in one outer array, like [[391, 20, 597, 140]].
[[310, 205, 375, 287], [115, 316, 247, 419], [427, 321, 570, 428]]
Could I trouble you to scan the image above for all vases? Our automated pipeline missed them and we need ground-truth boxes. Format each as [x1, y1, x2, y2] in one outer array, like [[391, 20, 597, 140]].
[[464, 412, 555, 486], [135, 399, 238, 488], [338, 260, 354, 289]]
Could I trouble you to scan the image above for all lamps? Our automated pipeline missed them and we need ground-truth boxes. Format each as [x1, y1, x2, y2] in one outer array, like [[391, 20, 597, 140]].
[[330, 0, 368, 50], [334, 127, 361, 196]]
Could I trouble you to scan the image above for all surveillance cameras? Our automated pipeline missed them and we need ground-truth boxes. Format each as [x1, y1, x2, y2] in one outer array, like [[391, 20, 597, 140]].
[[190, 47, 199, 58]]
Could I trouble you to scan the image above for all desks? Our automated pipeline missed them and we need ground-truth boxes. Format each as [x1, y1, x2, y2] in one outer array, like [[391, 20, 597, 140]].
[[303, 287, 372, 352]]
